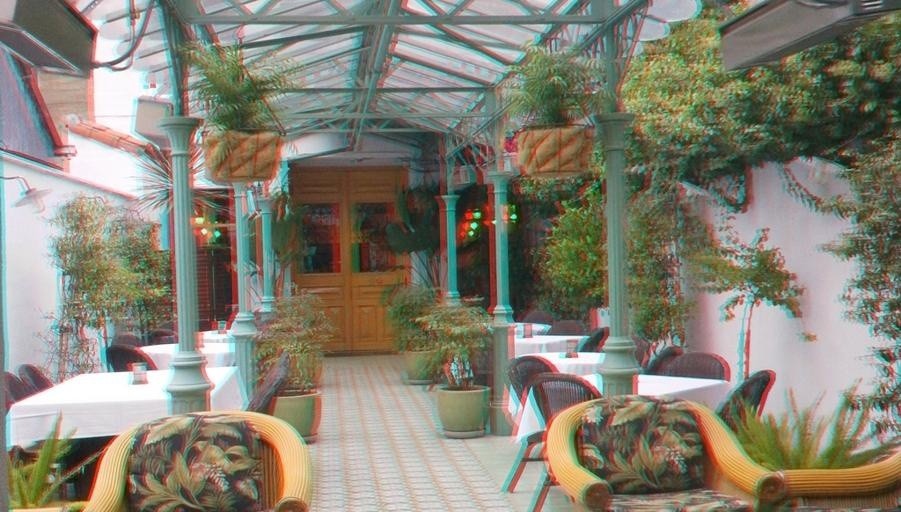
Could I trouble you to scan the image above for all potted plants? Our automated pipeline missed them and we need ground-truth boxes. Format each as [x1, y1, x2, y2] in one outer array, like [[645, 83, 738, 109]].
[[495, 34, 620, 181], [168, 33, 312, 183]]
